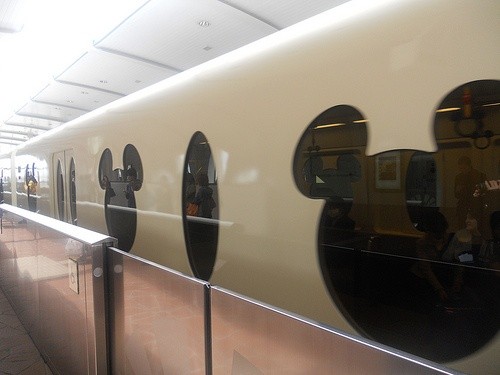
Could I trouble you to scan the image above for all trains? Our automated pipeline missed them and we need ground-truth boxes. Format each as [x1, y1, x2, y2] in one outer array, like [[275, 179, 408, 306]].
[[0, 0, 500, 375]]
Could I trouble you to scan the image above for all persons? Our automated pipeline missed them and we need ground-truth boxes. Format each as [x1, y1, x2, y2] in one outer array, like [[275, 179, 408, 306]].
[[443, 205, 486, 284], [183, 167, 216, 228], [101, 175, 115, 208], [479, 210, 500, 280], [403, 213, 457, 310], [124, 167, 142, 235], [323, 196, 357, 253]]
